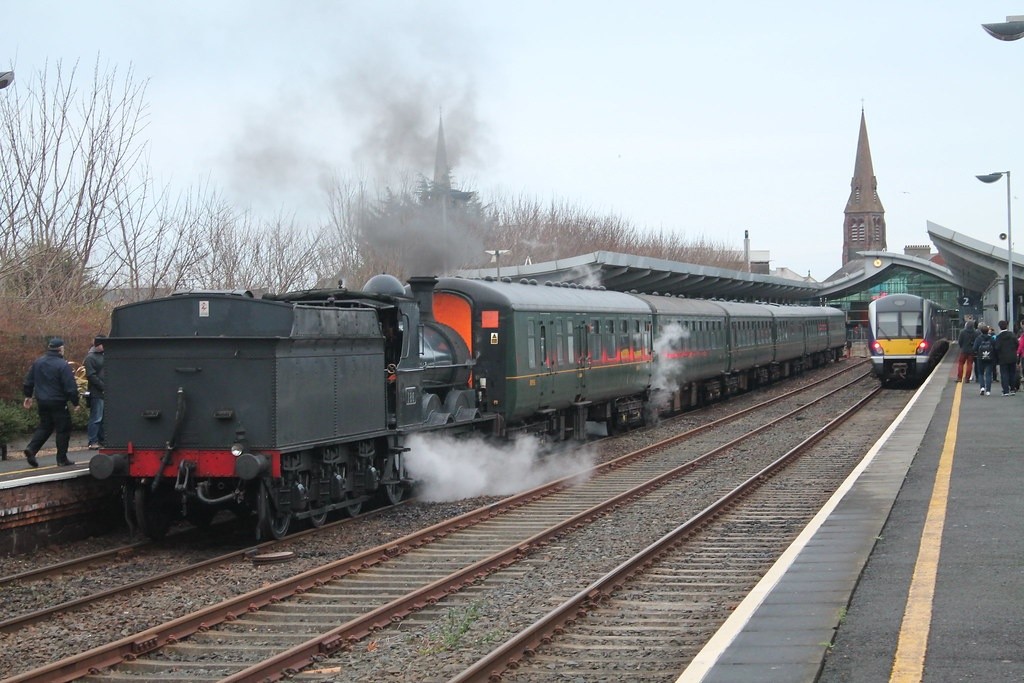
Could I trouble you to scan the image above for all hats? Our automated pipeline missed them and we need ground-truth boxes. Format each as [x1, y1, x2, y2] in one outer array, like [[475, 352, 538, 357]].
[[94, 336, 105, 347], [49, 338, 64, 348]]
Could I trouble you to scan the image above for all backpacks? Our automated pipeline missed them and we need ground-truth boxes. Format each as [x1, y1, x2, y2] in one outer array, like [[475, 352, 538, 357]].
[[979, 335, 993, 360]]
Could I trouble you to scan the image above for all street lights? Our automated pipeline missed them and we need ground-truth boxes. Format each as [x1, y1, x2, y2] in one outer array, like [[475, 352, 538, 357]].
[[975, 169, 1015, 332], [440, 190, 474, 278], [484, 248, 513, 282]]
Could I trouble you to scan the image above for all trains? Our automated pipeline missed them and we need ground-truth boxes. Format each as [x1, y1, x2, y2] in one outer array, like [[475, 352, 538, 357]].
[[867, 293, 950, 388], [88, 268, 848, 541]]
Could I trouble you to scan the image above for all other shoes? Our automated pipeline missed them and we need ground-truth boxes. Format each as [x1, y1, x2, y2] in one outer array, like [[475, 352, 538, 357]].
[[1011, 390, 1015, 395], [980, 387, 985, 396], [57, 457, 75, 466], [89, 442, 105, 450], [986, 391, 990, 395], [955, 379, 961, 383], [1004, 392, 1010, 396], [24, 447, 38, 467], [965, 379, 970, 383]]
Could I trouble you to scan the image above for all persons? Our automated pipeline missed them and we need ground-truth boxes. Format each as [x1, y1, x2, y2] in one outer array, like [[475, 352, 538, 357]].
[[954, 320, 977, 383], [23, 339, 79, 467], [973, 326, 995, 396], [1016, 320, 1024, 383], [85, 335, 105, 449], [994, 321, 1019, 396], [978, 322, 999, 382]]
